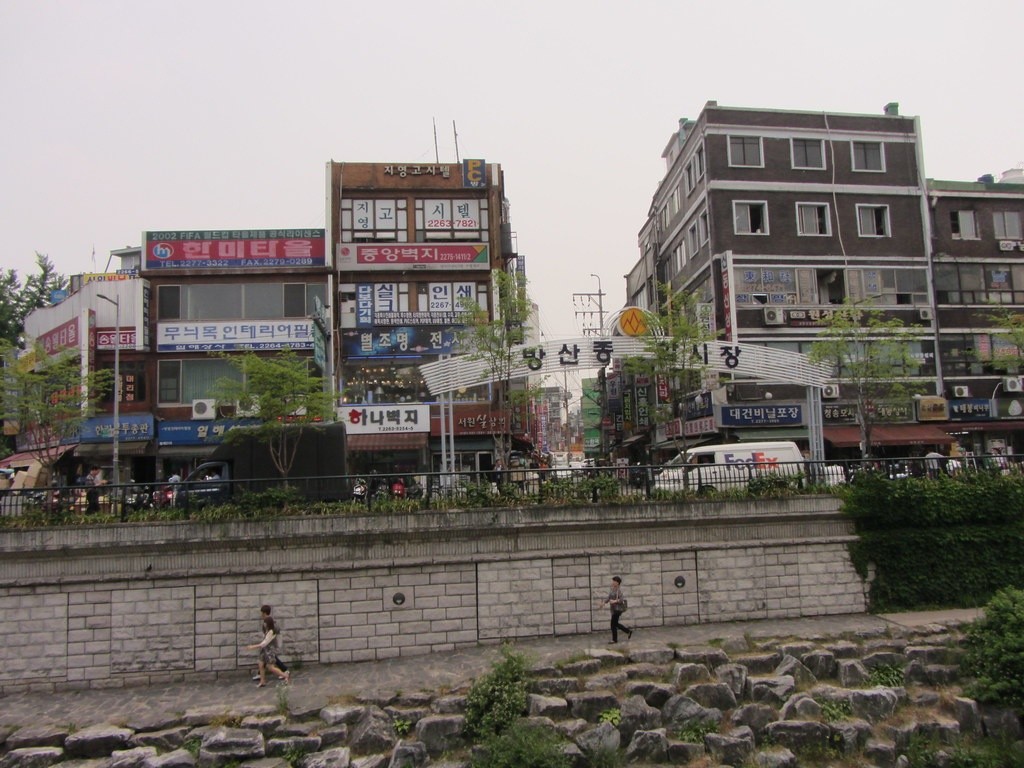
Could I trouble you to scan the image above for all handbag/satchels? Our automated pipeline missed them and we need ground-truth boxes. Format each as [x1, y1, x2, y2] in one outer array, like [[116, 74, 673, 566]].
[[613, 590, 628, 611]]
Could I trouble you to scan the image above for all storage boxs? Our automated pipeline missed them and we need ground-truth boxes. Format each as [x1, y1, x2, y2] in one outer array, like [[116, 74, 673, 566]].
[[13, 470, 28, 482], [0, 480, 10, 490], [10, 482, 23, 495], [24, 476, 43, 488], [27, 464, 48, 480], [0, 474, 6, 479], [34, 456, 50, 465]]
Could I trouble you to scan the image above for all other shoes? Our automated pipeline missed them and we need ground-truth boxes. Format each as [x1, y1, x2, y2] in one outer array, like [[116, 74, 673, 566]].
[[258, 683, 266, 688], [608, 640, 616, 644], [628, 631, 633, 638], [252, 675, 261, 680], [279, 670, 290, 679], [283, 675, 289, 685]]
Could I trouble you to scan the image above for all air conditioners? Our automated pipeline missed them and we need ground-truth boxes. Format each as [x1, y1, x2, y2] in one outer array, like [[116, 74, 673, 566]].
[[282, 394, 308, 416], [919, 308, 933, 321], [952, 385, 969, 398], [1003, 377, 1023, 392], [234, 394, 262, 416], [762, 306, 785, 324], [192, 397, 217, 419], [822, 383, 840, 400]]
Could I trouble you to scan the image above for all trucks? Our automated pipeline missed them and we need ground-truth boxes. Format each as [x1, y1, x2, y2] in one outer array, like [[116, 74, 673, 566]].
[[170, 421, 348, 514]]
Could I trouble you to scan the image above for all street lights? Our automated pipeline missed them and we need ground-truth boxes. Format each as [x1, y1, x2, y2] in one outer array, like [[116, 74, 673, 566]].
[[95, 292, 120, 517]]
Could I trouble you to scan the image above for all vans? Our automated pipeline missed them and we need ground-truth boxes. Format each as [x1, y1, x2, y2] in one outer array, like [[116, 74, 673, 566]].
[[650, 441, 808, 497]]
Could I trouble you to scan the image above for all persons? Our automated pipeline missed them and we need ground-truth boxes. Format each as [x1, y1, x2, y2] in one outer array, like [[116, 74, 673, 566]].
[[949, 440, 966, 477], [247, 617, 289, 688], [597, 575, 633, 645], [205, 469, 220, 480], [588, 469, 595, 479], [919, 448, 950, 480], [82, 464, 109, 514], [490, 459, 502, 484], [992, 447, 1006, 471], [369, 468, 380, 499], [253, 604, 290, 680], [6, 464, 11, 469]]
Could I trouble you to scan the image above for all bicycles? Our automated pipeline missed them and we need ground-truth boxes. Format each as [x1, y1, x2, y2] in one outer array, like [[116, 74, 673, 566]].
[[426, 479, 469, 507]]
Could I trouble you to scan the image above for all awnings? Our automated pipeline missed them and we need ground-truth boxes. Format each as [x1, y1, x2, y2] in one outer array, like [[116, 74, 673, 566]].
[[822, 426, 956, 447], [530, 447, 539, 458], [0, 442, 76, 469], [731, 428, 810, 442], [513, 432, 531, 448], [622, 434, 644, 448], [74, 440, 150, 457], [345, 432, 430, 450], [644, 437, 711, 450], [160, 447, 220, 456]]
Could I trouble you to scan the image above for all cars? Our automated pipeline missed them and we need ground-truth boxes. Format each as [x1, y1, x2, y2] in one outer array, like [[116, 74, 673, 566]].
[[789, 458, 912, 491]]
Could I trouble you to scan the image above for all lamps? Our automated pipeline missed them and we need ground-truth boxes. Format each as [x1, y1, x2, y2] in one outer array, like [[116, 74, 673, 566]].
[[373, 386, 385, 402]]
